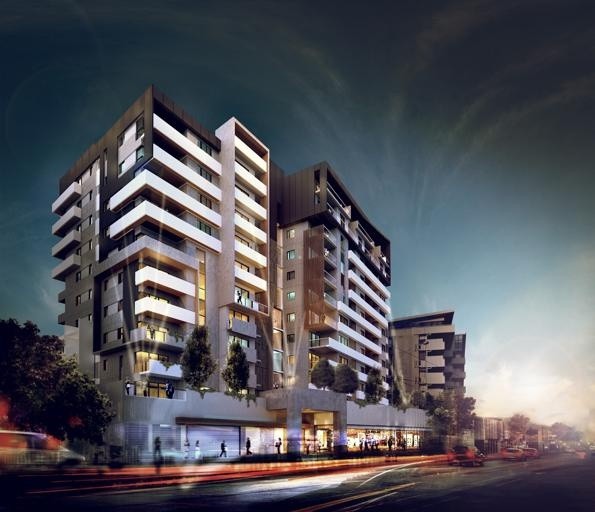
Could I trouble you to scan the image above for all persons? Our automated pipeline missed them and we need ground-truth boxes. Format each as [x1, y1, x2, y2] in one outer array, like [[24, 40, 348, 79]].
[[194, 440, 201, 458], [153, 436, 163, 458], [245, 437, 253, 455], [358, 438, 407, 451], [277, 438, 282, 454], [219, 439, 228, 457], [183, 437, 191, 458], [164, 379, 175, 399], [236, 290, 242, 304]]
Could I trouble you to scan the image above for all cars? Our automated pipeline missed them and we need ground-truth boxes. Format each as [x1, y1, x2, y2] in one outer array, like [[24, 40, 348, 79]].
[[502, 448, 524, 462], [447, 445, 484, 467], [523, 448, 539, 459]]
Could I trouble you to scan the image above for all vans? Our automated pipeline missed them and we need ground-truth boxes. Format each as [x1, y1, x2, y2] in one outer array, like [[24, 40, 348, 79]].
[[0, 430, 86, 470]]
